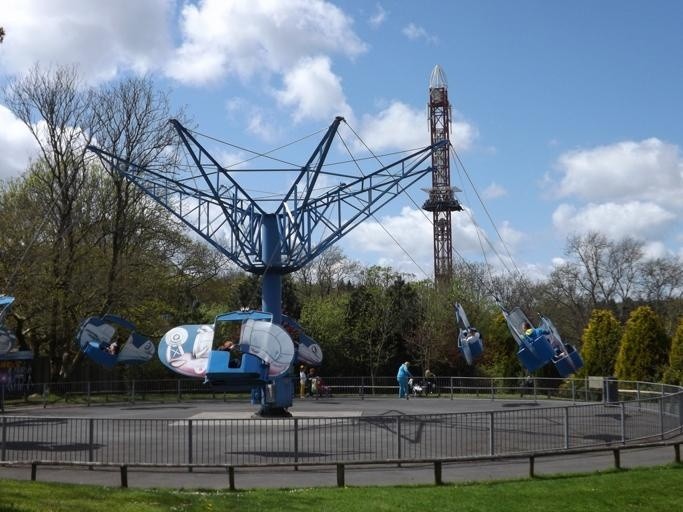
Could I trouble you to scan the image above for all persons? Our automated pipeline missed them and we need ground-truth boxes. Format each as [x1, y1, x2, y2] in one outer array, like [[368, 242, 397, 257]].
[[425, 370, 436, 393], [306, 368, 318, 400], [396, 361, 415, 399], [298, 365, 305, 398], [519, 324, 553, 351]]
